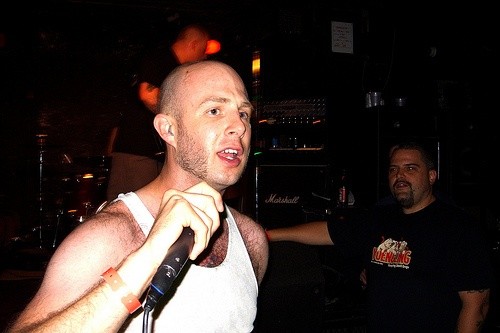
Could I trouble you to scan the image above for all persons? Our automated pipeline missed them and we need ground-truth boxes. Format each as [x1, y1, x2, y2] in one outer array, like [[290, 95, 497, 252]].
[[264, 133, 495, 333], [6, 59, 269, 333], [106, 21, 211, 203]]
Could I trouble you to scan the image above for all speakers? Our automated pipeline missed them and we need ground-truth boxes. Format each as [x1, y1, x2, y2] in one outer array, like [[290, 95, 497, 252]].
[[256, 150, 330, 246]]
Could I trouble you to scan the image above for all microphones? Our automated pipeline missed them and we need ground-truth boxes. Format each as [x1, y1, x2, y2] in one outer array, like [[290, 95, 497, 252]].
[[145, 227, 195, 311]]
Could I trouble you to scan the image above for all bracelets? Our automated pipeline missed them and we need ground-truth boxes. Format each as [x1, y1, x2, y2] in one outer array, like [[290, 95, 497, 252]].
[[101, 267, 142, 314]]
